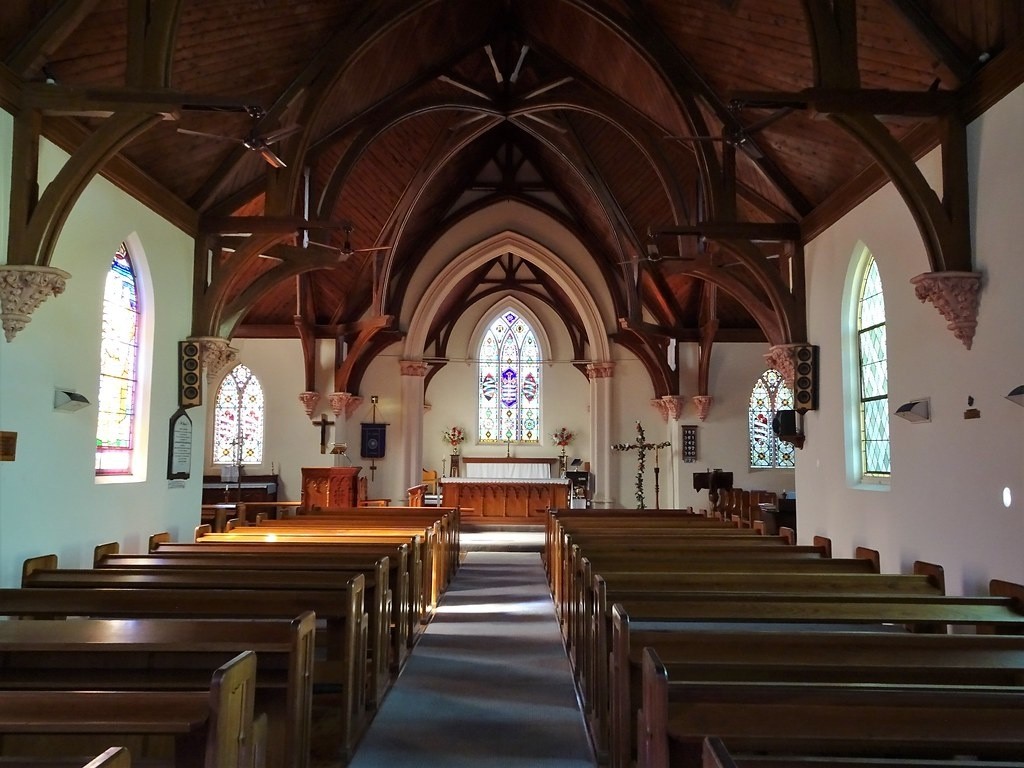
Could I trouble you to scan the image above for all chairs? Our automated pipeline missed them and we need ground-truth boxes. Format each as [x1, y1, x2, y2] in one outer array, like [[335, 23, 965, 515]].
[[422, 467, 438, 495], [358, 476, 391, 506]]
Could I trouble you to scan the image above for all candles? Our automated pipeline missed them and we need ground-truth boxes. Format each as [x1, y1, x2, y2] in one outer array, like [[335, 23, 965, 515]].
[[225, 485, 228, 491], [655, 444, 658, 468]]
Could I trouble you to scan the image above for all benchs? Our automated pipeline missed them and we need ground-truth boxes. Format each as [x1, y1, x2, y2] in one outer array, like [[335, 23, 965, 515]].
[[0, 504, 461, 768], [540, 507, 1024, 768], [201, 504, 236, 533]]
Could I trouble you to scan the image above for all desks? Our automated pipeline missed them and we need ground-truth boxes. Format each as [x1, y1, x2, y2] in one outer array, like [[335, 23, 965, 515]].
[[459, 458, 561, 478], [217, 501, 301, 505], [437, 478, 574, 525]]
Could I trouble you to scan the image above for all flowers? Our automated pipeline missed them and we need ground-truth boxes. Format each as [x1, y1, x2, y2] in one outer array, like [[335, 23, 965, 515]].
[[544, 424, 584, 448], [440, 425, 465, 447]]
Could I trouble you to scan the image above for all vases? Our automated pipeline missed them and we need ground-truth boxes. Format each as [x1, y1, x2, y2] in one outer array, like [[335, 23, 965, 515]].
[[560, 446, 566, 456], [453, 446, 458, 455]]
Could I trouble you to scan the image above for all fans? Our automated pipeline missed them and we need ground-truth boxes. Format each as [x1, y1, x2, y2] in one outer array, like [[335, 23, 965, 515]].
[[177, 106, 306, 168], [661, 99, 795, 160], [618, 233, 696, 264], [306, 226, 392, 262]]
